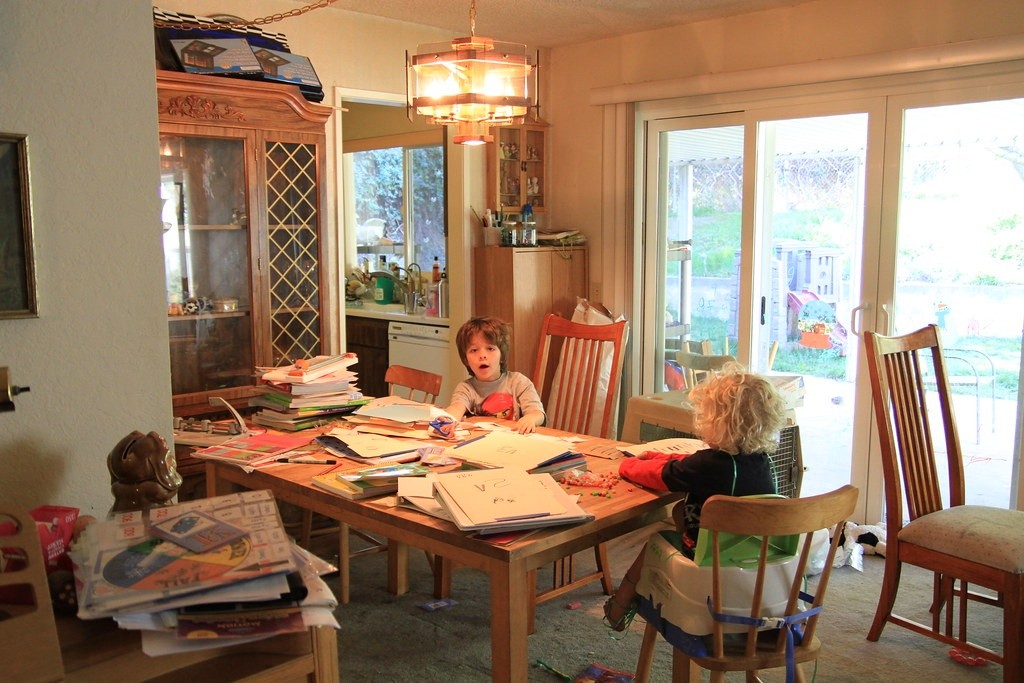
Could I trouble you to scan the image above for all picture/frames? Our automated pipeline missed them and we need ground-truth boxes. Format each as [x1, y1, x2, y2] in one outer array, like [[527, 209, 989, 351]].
[[0, 131, 39, 321]]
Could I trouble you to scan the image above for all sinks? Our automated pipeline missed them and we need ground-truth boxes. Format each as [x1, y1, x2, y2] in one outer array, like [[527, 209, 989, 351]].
[[350, 303, 425, 314]]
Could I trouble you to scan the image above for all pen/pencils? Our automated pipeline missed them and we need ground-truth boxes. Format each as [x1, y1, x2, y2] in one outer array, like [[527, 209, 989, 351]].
[[315, 463, 343, 476], [500, 204, 503, 222], [277, 458, 336, 464], [537, 659, 571, 680], [495, 211, 499, 226], [470, 205, 484, 227], [505, 212, 510, 221]]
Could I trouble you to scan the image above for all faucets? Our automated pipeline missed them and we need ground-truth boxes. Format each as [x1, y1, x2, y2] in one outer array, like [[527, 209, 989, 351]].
[[369, 270, 418, 314], [407, 263, 422, 296]]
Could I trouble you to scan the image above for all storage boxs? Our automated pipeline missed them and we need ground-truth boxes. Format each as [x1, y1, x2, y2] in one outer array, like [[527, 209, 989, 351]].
[[768, 376, 806, 409]]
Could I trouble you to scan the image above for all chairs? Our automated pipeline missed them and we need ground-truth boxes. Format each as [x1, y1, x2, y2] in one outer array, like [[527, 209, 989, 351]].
[[917, 348, 995, 445], [434, 314, 630, 635], [385, 364, 441, 405], [634, 485, 859, 683], [665, 339, 737, 391], [864, 322, 1024, 683]]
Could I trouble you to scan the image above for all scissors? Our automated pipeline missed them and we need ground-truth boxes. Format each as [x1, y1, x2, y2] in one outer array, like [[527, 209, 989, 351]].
[[416, 416, 454, 436]]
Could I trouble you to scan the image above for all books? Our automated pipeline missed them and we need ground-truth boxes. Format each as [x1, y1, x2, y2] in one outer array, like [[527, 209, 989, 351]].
[[249, 351, 376, 432], [538, 228, 580, 240], [191, 395, 596, 534]]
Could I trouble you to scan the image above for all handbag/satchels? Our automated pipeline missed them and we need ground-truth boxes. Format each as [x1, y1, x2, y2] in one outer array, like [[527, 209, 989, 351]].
[[537, 231, 587, 259]]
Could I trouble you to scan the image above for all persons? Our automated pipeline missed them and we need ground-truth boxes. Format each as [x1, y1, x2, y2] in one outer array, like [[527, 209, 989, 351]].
[[603, 372, 785, 632], [439, 317, 547, 434]]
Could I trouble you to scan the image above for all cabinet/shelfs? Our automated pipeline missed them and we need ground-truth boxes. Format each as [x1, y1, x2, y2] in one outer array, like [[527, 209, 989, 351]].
[[665, 164, 692, 344], [474, 245, 589, 412], [156, 68, 347, 415], [489, 113, 550, 214]]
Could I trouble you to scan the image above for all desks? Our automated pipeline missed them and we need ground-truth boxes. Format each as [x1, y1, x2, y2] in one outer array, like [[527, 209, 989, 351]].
[[203, 416, 685, 683], [0, 601, 338, 683]]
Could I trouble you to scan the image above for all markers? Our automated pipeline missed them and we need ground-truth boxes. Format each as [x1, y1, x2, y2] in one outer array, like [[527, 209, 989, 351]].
[[487, 209, 492, 227]]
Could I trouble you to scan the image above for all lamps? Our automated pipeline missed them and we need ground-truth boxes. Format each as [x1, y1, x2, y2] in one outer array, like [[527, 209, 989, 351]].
[[405, 0, 541, 147]]
